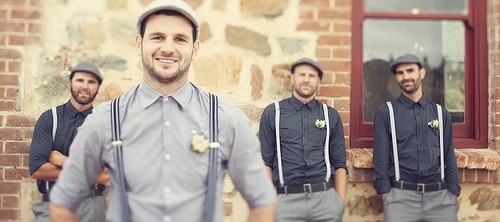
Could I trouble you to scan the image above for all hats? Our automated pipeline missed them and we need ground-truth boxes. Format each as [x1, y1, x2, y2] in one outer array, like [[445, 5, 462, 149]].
[[137, 0, 201, 42], [69, 64, 103, 83], [291, 58, 323, 80], [389, 55, 422, 74]]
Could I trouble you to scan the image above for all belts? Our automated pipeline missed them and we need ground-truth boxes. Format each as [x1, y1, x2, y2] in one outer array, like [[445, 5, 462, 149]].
[[390, 181, 448, 194], [277, 177, 333, 195], [43, 189, 103, 201]]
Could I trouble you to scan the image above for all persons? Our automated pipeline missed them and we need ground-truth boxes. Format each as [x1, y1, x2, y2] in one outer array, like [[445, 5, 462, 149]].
[[48, 0, 278, 222], [373, 55, 461, 222], [28, 62, 110, 222], [259, 58, 348, 222]]
